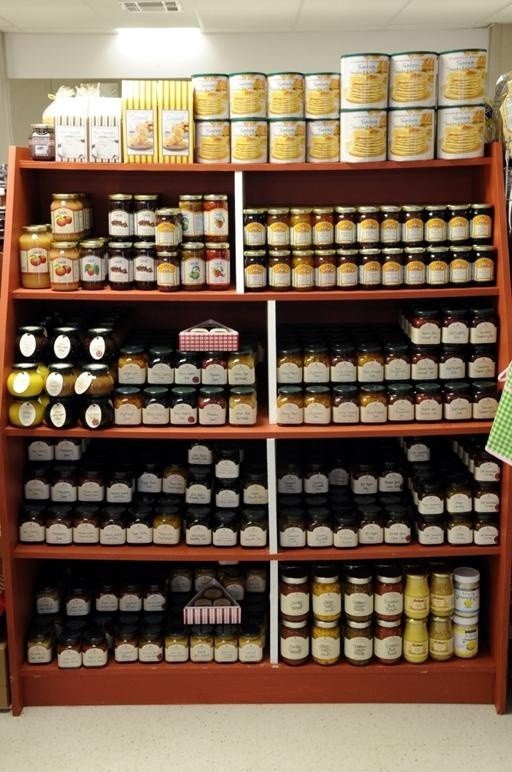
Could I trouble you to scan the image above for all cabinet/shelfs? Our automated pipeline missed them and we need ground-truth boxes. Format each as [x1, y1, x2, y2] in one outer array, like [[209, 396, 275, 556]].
[[2, 143, 511, 716]]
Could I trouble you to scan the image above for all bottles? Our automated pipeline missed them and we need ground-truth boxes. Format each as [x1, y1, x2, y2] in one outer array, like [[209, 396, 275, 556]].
[[108, 243, 133, 291], [344, 571, 374, 622], [356, 207, 379, 249], [378, 462, 402, 492], [133, 195, 156, 241], [29, 613, 170, 632], [26, 438, 54, 462], [74, 506, 100, 544], [34, 581, 60, 615], [455, 618, 478, 659], [279, 620, 309, 666], [312, 572, 342, 622], [473, 245, 496, 287], [144, 585, 166, 613], [14, 326, 48, 364], [240, 511, 267, 548], [474, 483, 500, 514], [398, 206, 423, 247], [50, 193, 80, 242], [205, 242, 230, 291], [268, 251, 292, 292], [155, 209, 178, 251], [449, 246, 472, 289], [267, 208, 289, 250], [26, 626, 53, 664], [212, 512, 237, 547], [446, 480, 472, 516], [474, 516, 498, 546], [357, 341, 384, 383], [437, 346, 466, 382], [32, 302, 139, 336], [82, 365, 114, 398], [381, 248, 403, 290], [403, 620, 429, 663], [56, 634, 82, 668], [113, 387, 141, 425], [409, 310, 440, 346], [330, 459, 349, 485], [106, 467, 132, 503], [473, 451, 498, 482], [448, 204, 471, 245], [19, 225, 50, 289], [238, 628, 263, 664], [83, 630, 108, 667], [78, 463, 103, 502], [430, 571, 454, 618], [80, 399, 114, 430], [470, 203, 493, 245], [441, 310, 469, 346], [72, 189, 93, 239], [360, 385, 387, 424], [139, 626, 163, 664], [278, 459, 301, 493], [281, 560, 454, 569], [203, 195, 228, 241], [142, 386, 169, 426], [310, 623, 342, 666], [174, 349, 201, 385], [429, 617, 454, 661], [415, 383, 442, 423], [335, 250, 358, 290], [163, 462, 185, 495], [134, 242, 156, 291], [45, 364, 78, 397], [380, 206, 400, 248], [417, 484, 444, 515], [100, 507, 125, 545], [359, 248, 381, 290], [394, 303, 416, 336], [7, 363, 43, 398], [278, 507, 306, 548], [157, 251, 179, 292], [214, 481, 239, 508], [222, 569, 245, 604], [350, 462, 377, 495], [243, 209, 266, 250], [188, 440, 214, 466], [190, 631, 213, 663], [48, 241, 80, 291], [443, 382, 472, 423], [55, 438, 81, 461], [170, 564, 192, 592], [306, 509, 332, 547], [384, 508, 410, 544], [453, 567, 480, 618], [147, 347, 174, 385], [358, 507, 382, 544], [453, 441, 465, 457], [50, 467, 77, 502], [170, 387, 198, 424], [427, 247, 450, 288], [114, 629, 138, 663], [303, 341, 330, 384], [403, 247, 425, 288], [243, 471, 267, 507], [289, 207, 312, 250], [95, 581, 118, 613], [185, 508, 211, 547], [66, 586, 91, 617], [463, 448, 468, 465], [201, 352, 227, 385], [45, 398, 79, 428], [194, 567, 216, 592], [244, 250, 267, 292], [342, 621, 374, 665], [8, 399, 45, 428], [214, 626, 237, 664], [311, 207, 334, 249], [127, 504, 152, 545], [418, 517, 444, 545], [374, 620, 403, 664], [216, 447, 239, 479], [314, 250, 336, 290], [407, 437, 430, 462], [253, 334, 265, 363], [48, 327, 80, 363], [304, 387, 331, 425], [108, 194, 133, 241], [332, 385, 359, 424], [472, 381, 499, 420], [469, 309, 497, 346], [304, 458, 329, 493], [467, 346, 496, 381], [228, 387, 257, 426], [179, 195, 204, 241], [292, 249, 314, 291], [123, 325, 179, 346], [278, 569, 310, 622], [198, 387, 226, 424], [276, 386, 304, 426], [468, 452, 475, 474], [257, 381, 268, 406], [385, 343, 410, 381], [388, 384, 415, 424], [136, 462, 163, 494], [244, 567, 266, 594], [335, 207, 356, 249], [118, 585, 142, 612], [406, 469, 424, 506], [330, 345, 356, 383], [332, 513, 358, 547], [411, 347, 438, 383], [403, 572, 430, 619], [447, 516, 473, 546], [24, 463, 49, 501], [186, 469, 211, 505], [373, 573, 404, 621], [82, 328, 117, 364], [152, 506, 180, 546], [164, 625, 188, 663], [79, 241, 105, 289], [118, 346, 146, 386], [425, 205, 446, 247], [19, 503, 46, 543], [281, 492, 403, 509], [276, 343, 303, 385], [46, 506, 73, 544], [228, 350, 255, 386], [28, 124, 56, 161], [182, 242, 204, 291]]
